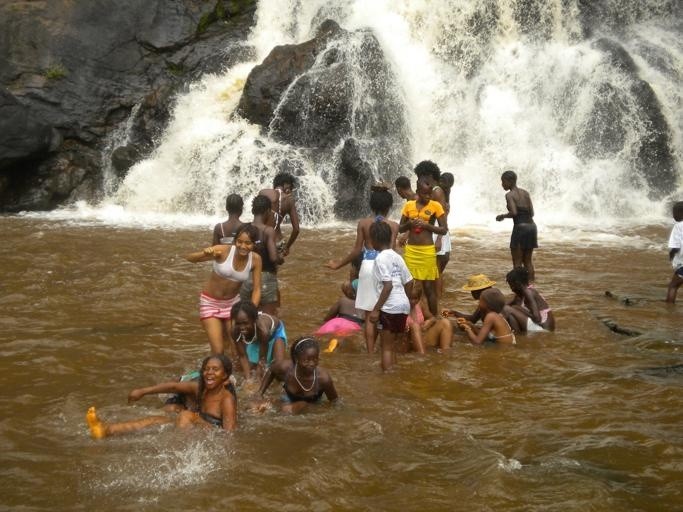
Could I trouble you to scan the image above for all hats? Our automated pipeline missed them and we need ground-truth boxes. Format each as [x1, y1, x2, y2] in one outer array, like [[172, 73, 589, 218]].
[[462, 274, 496, 291]]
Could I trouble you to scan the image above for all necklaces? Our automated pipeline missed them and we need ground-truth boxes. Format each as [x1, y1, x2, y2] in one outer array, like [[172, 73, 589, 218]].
[[293, 363, 316, 392]]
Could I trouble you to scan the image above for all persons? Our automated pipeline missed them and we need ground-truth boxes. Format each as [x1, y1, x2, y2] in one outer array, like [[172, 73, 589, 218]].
[[186, 223, 262, 356], [313, 279, 367, 339], [230, 300, 287, 379], [413, 159, 446, 253], [442, 275, 497, 327], [496, 171, 539, 285], [235, 194, 285, 314], [213, 193, 246, 248], [327, 190, 401, 299], [399, 179, 447, 317], [394, 176, 416, 202], [259, 173, 299, 257], [501, 267, 555, 336], [665, 201, 683, 304], [369, 221, 413, 370], [254, 337, 338, 413], [457, 289, 516, 350], [402, 278, 452, 355], [87, 353, 236, 441], [439, 171, 454, 206]]
